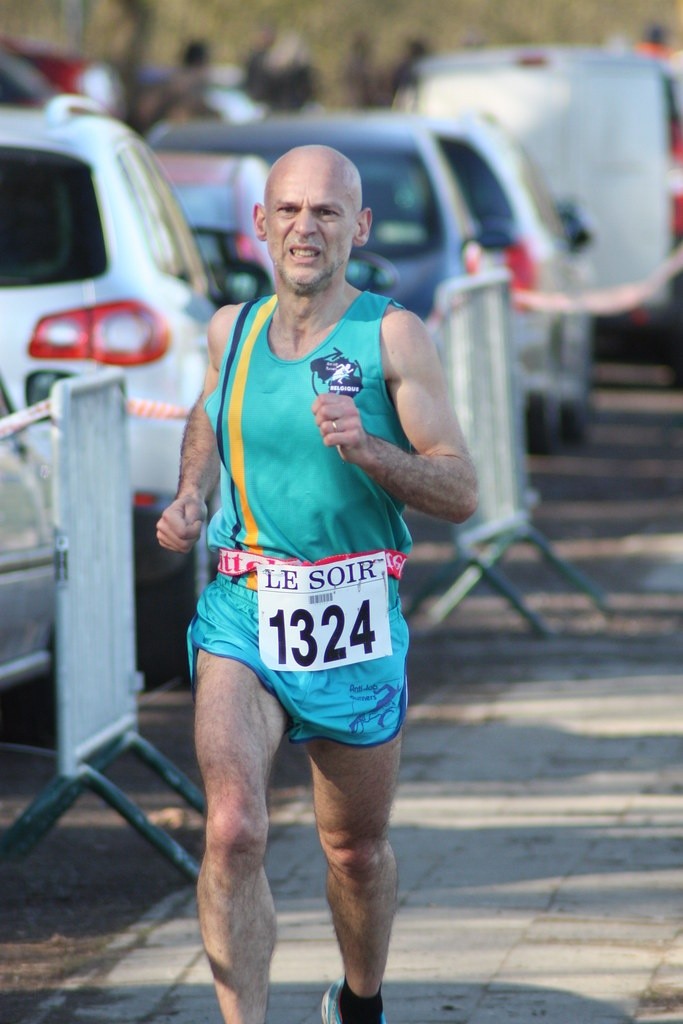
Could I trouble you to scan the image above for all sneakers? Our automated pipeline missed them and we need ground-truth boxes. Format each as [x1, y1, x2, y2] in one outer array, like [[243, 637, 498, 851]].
[[322, 976, 387, 1024]]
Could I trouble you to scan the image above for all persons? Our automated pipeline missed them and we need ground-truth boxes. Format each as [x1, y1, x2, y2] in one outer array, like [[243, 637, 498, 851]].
[[155, 145, 479, 1024], [107, 1, 669, 140]]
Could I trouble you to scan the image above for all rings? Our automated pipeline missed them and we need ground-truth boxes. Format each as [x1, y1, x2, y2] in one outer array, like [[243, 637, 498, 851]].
[[332, 420, 337, 433]]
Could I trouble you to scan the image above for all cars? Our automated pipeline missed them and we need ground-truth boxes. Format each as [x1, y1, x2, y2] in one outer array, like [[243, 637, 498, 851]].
[[148, 46, 683, 584]]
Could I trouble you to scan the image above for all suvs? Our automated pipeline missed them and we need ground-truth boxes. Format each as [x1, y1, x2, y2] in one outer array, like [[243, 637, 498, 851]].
[[0, 88, 235, 701]]
